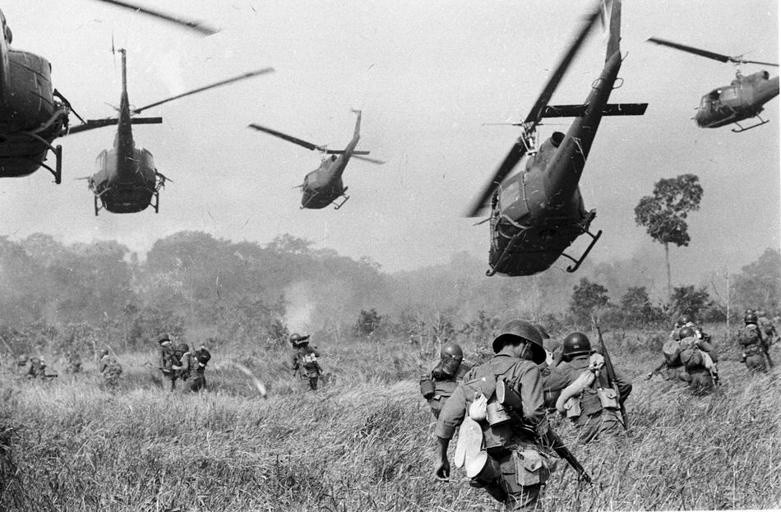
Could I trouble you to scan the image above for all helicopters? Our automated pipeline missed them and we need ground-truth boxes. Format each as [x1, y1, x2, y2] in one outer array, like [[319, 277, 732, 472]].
[[249, 108, 385, 210], [62, 34, 277, 216], [646, 37, 779, 133], [460, 0, 649, 276], [0, 0, 216, 184]]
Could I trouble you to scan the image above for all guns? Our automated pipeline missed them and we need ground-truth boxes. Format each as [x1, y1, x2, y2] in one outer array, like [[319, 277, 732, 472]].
[[596, 326, 629, 429], [496, 380, 592, 483], [755, 327, 773, 369]]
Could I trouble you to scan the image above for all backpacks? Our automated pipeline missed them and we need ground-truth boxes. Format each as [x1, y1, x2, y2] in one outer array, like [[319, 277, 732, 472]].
[[680, 344, 702, 367], [739, 325, 758, 344]]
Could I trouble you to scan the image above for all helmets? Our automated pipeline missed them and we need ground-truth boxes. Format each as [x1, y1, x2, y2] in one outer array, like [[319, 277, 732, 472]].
[[745, 314, 756, 323], [290, 334, 299, 343], [441, 344, 462, 362], [492, 320, 546, 365], [100, 348, 108, 358], [680, 327, 693, 338], [560, 332, 591, 358], [159, 333, 169, 344], [176, 344, 188, 353]]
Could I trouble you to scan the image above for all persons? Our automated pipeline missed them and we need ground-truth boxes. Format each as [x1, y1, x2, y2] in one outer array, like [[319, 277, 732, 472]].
[[173, 343, 210, 393], [533, 324, 564, 416], [542, 332, 632, 443], [27, 356, 57, 383], [737, 308, 774, 375], [289, 333, 323, 391], [99, 349, 124, 395], [433, 320, 554, 510], [658, 318, 718, 395], [158, 335, 179, 390], [63, 352, 83, 377], [420, 343, 463, 419]]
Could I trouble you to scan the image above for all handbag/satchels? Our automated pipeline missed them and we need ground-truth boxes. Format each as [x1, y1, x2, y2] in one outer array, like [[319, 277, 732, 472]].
[[514, 449, 543, 486], [564, 398, 580, 417], [597, 387, 620, 409]]
